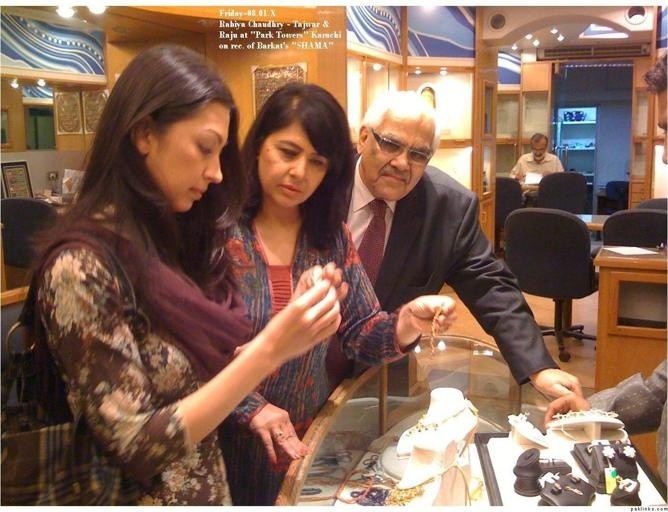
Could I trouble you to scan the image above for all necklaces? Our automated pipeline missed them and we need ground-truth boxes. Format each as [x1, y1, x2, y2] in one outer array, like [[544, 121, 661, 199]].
[[416, 398, 467, 432], [384, 461, 454, 506]]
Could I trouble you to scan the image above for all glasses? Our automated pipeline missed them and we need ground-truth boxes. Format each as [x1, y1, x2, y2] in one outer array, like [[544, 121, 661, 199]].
[[370, 127, 430, 163]]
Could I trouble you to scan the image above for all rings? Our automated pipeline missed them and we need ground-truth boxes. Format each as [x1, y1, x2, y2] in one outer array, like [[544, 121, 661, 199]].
[[273, 431, 284, 440]]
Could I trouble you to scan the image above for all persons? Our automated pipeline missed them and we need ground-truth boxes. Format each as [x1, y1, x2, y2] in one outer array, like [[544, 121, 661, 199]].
[[544, 50, 668, 491], [209, 81, 455, 507], [18, 46, 348, 506], [335, 89, 581, 399], [510, 133, 565, 205]]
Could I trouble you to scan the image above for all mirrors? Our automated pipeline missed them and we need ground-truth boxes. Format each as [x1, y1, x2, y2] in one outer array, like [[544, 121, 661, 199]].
[[2, 78, 57, 152]]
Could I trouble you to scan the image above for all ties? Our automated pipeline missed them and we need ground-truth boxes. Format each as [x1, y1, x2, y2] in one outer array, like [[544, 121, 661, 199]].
[[357, 199, 386, 288]]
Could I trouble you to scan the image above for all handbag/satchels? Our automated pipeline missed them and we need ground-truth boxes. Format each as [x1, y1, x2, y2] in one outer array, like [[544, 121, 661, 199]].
[[1, 236, 149, 506]]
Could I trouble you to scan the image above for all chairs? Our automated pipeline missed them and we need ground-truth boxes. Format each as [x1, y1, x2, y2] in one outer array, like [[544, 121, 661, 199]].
[[492, 169, 668, 362]]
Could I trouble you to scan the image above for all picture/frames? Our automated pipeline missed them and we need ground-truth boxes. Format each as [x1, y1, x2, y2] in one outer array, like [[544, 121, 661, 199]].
[[0, 161, 34, 202], [251, 63, 308, 127], [1, 104, 15, 149]]
